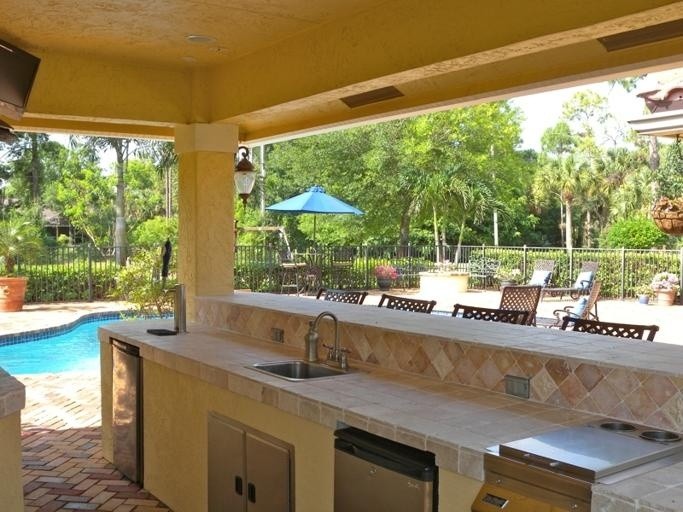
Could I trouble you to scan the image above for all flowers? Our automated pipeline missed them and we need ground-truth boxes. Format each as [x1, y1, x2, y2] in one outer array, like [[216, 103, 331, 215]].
[[651, 272, 681, 296], [374, 265, 399, 280]]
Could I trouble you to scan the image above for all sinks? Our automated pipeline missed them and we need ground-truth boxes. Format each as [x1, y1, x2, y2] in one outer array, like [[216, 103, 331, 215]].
[[244, 359, 355, 383]]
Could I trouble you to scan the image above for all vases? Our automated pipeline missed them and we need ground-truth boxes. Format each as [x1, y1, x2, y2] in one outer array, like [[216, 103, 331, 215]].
[[377, 278, 392, 291], [654, 289, 676, 305]]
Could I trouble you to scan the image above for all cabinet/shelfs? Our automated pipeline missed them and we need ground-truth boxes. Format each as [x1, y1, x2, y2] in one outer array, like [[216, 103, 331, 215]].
[[207, 410, 295, 512]]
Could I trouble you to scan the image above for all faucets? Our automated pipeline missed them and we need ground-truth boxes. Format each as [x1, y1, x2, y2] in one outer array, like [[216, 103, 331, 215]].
[[313, 312, 340, 362]]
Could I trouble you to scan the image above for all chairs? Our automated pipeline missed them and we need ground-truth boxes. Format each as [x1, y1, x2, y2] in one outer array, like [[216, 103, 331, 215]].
[[562, 316, 660, 342], [273, 245, 354, 297], [316, 286, 540, 328], [526, 259, 603, 329]]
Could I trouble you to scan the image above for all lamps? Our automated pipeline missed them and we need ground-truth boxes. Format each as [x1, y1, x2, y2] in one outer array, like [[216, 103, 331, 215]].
[[235, 146, 255, 211]]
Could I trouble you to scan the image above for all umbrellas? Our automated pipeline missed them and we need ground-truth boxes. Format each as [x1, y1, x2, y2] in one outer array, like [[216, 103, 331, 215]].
[[264, 185, 365, 248]]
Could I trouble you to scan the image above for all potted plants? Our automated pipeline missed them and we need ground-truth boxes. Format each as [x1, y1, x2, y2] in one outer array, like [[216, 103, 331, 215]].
[[0, 202, 51, 313], [494, 265, 526, 297], [634, 284, 652, 304]]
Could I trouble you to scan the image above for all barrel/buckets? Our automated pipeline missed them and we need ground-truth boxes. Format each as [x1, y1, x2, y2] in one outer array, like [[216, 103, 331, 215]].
[[1, 275, 28, 311]]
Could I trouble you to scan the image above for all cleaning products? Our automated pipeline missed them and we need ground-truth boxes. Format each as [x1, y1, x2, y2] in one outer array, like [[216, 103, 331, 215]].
[[305, 320, 320, 362]]
